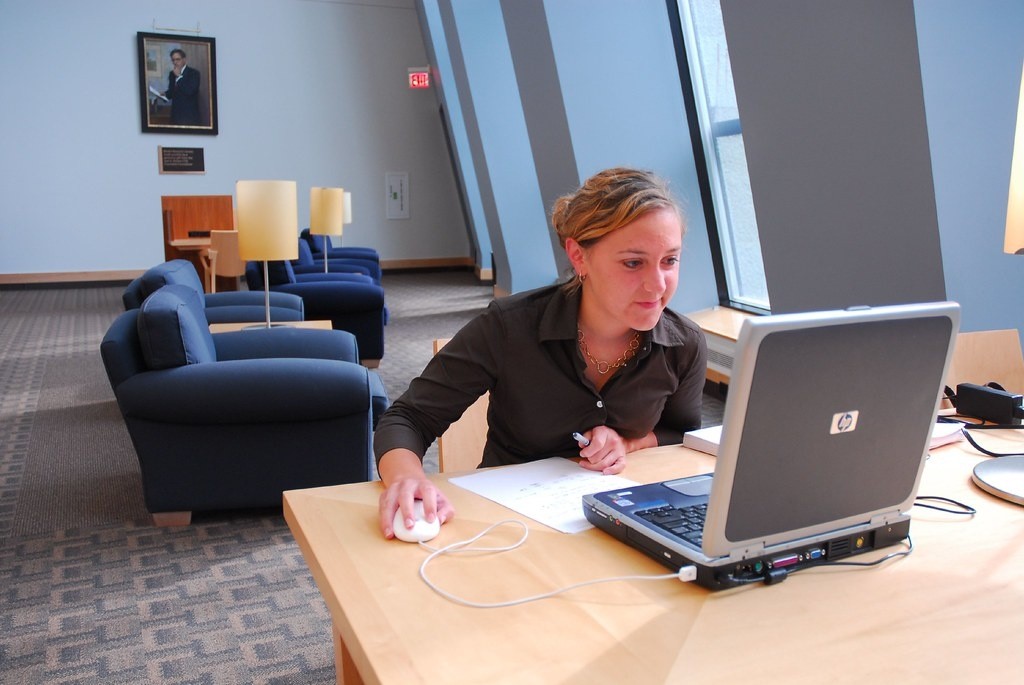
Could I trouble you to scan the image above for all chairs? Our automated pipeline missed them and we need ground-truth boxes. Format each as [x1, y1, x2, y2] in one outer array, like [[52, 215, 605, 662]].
[[123, 258, 305, 325], [244, 259, 387, 369], [199, 229, 248, 295], [433, 336, 489, 472], [100, 282, 389, 526], [302, 229, 380, 264], [940, 330, 1024, 409], [289, 238, 383, 285]]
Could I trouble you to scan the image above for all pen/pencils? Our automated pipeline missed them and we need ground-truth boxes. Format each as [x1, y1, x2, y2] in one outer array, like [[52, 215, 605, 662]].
[[573, 432, 590, 446]]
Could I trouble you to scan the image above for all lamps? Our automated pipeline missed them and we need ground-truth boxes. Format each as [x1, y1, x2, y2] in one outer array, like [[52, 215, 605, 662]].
[[235, 179, 300, 331], [308, 186, 345, 274], [340, 192, 353, 248]]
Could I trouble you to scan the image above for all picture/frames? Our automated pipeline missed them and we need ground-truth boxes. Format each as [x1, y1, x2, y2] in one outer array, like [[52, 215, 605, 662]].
[[137, 32, 220, 137]]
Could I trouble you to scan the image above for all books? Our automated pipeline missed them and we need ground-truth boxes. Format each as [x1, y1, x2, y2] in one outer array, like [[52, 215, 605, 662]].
[[684, 423, 969, 458], [149, 85, 169, 104]]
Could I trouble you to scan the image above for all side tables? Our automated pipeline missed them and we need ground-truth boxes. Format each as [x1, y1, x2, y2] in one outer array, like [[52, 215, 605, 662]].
[[208, 320, 333, 333]]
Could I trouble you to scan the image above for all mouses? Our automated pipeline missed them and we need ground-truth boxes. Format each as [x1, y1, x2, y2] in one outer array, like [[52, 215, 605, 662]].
[[395, 497, 440, 542]]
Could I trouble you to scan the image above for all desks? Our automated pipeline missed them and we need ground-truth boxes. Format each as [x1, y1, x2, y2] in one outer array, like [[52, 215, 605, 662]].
[[278, 402, 1024, 685], [167, 237, 211, 252]]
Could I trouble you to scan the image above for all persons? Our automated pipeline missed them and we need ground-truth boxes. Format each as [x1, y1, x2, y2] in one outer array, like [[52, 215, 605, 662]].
[[374, 166, 707, 541], [161, 49, 201, 126]]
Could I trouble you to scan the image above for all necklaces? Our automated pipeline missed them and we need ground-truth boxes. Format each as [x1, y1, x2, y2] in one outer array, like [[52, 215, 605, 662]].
[[576, 319, 641, 375]]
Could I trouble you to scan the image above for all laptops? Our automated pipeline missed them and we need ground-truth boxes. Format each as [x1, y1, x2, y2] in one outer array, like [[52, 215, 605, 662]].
[[583, 300, 960, 590]]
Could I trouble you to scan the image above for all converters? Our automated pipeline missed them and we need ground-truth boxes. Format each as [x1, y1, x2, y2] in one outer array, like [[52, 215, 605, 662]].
[[957, 382, 1023, 427]]
